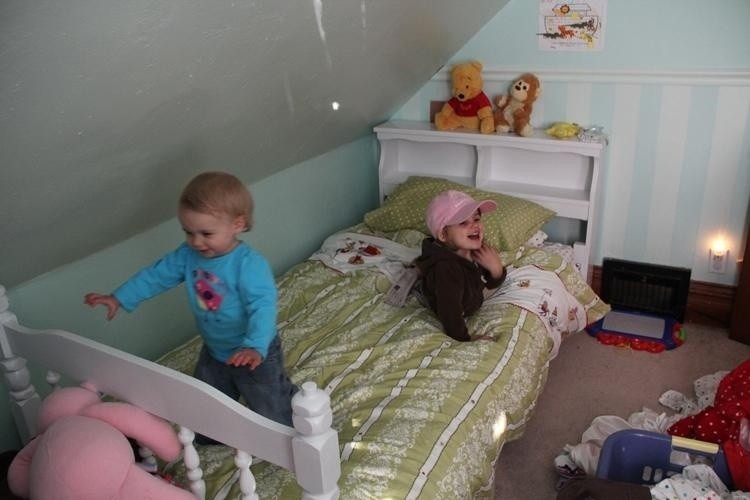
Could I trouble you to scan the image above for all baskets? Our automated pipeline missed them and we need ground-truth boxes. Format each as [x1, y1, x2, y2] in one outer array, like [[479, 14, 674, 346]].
[[595, 427, 736, 493]]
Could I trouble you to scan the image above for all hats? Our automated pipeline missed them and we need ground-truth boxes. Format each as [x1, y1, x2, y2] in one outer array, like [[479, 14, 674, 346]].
[[424, 190, 498, 241]]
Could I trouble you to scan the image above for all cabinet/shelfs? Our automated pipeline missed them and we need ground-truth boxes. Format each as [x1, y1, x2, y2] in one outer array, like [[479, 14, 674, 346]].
[[372, 116, 602, 286]]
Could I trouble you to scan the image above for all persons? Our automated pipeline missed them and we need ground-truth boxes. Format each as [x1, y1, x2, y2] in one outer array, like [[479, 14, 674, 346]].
[[409, 191, 507, 342], [83, 172, 300, 446]]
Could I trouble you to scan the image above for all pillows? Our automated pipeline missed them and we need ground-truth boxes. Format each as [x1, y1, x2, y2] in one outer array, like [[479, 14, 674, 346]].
[[363, 174, 557, 263]]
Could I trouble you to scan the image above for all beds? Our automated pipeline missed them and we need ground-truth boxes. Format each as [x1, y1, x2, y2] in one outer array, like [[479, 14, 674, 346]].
[[3, 214, 584, 500]]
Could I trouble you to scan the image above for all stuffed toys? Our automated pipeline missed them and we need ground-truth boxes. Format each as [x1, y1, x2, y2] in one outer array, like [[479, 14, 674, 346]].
[[7, 382, 198, 500], [435, 62, 495, 133], [545, 123, 577, 138], [493, 72, 541, 137]]
